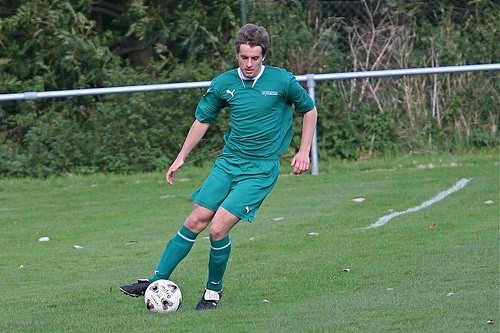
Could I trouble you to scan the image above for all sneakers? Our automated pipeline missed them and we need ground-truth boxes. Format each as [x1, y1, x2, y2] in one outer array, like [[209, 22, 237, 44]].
[[120, 278, 150, 298], [196, 289, 223, 312]]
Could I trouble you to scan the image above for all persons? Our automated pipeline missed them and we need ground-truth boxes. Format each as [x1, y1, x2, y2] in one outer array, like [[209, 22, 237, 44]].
[[120, 23, 317, 310]]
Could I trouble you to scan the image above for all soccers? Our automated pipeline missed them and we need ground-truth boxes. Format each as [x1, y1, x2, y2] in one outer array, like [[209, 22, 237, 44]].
[[145, 279, 182, 313]]
